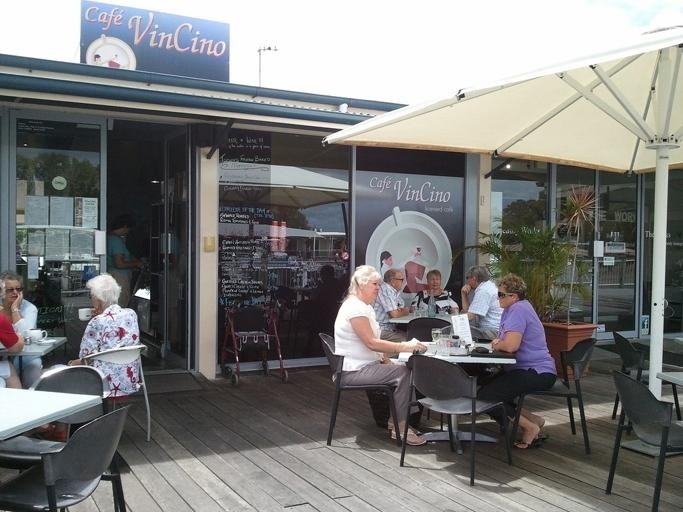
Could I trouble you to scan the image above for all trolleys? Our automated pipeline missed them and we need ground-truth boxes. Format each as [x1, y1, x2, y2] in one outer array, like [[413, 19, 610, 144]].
[[219, 304, 290, 389]]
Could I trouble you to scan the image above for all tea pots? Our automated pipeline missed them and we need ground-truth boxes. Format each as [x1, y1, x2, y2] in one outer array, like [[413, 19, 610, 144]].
[[24, 329, 48, 343]]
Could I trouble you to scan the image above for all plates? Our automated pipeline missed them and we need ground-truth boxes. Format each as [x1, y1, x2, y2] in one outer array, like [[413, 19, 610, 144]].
[[37, 340, 56, 344], [366, 210, 453, 292]]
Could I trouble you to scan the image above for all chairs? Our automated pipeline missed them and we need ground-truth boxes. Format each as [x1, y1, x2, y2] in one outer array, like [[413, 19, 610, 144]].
[[1, 403, 132, 512], [399, 351, 518, 487], [610, 330, 654, 417], [30, 365, 111, 406], [526, 337, 593, 449], [317, 329, 403, 449], [78, 344, 152, 441], [408, 316, 452, 345], [599, 369, 683, 510]]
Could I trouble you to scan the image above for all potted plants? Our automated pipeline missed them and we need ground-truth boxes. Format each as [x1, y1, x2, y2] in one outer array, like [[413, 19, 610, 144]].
[[537, 186, 599, 383]]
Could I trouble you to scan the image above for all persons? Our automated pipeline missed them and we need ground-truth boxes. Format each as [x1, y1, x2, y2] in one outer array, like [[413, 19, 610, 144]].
[[35, 272, 142, 440], [0, 287, 50, 436], [107, 217, 143, 307], [0, 270, 42, 389], [330, 265, 556, 449], [381, 251, 393, 278]]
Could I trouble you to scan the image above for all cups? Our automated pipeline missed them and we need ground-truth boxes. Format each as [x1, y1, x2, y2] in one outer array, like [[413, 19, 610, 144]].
[[416, 308, 444, 318], [425, 328, 450, 356], [270, 221, 287, 252], [377, 207, 441, 283], [78, 308, 92, 321]]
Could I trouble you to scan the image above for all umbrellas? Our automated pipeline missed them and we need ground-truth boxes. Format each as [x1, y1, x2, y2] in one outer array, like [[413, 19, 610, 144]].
[[320, 26, 682, 458]]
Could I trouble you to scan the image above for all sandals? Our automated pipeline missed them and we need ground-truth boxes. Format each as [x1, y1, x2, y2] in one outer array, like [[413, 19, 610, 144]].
[[387, 417, 422, 436], [391, 428, 427, 446]]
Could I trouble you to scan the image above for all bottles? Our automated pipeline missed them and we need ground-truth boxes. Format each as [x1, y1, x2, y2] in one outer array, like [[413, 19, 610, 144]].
[[451, 336, 460, 348], [429, 291, 436, 318]]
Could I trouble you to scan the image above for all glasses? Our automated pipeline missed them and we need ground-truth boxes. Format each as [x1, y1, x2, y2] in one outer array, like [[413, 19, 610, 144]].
[[6, 287, 24, 293], [395, 278, 404, 282], [88, 291, 93, 299], [497, 291, 514, 299]]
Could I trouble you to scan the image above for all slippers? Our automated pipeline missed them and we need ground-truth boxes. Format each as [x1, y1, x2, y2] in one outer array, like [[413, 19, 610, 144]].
[[535, 433, 550, 441], [513, 439, 543, 450]]
